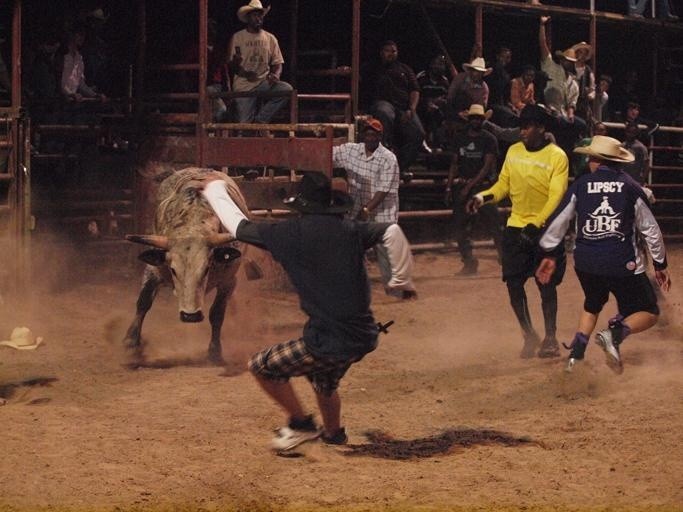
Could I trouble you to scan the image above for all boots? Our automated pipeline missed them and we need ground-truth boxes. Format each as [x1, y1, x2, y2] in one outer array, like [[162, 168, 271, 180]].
[[562, 331, 591, 373], [595, 319, 630, 377]]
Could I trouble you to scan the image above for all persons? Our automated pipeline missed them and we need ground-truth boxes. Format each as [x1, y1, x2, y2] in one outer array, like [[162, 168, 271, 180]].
[[466, 104, 569, 359], [199, 173, 418, 455], [0, 0, 671, 301], [535, 135, 671, 376]]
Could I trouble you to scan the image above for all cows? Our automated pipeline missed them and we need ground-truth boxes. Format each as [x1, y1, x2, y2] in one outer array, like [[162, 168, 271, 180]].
[[117, 160, 264, 377]]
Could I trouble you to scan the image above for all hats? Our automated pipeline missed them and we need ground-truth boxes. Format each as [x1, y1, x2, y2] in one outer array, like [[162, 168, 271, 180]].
[[512, 103, 548, 127], [462, 56, 493, 78], [279, 171, 353, 214], [459, 102, 489, 118], [570, 41, 593, 62], [356, 115, 384, 137], [236, 0, 271, 23], [556, 48, 578, 64], [571, 133, 637, 163], [0, 326, 42, 350]]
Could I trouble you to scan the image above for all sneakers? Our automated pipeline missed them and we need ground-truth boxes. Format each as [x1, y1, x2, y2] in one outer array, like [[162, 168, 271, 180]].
[[385, 283, 418, 301], [456, 254, 478, 277], [401, 170, 414, 181], [520, 333, 540, 359], [315, 427, 348, 446], [252, 120, 271, 139], [268, 414, 320, 452], [538, 333, 560, 359]]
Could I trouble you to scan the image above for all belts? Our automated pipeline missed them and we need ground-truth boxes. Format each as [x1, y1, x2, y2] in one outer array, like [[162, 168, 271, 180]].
[[458, 177, 496, 186]]
